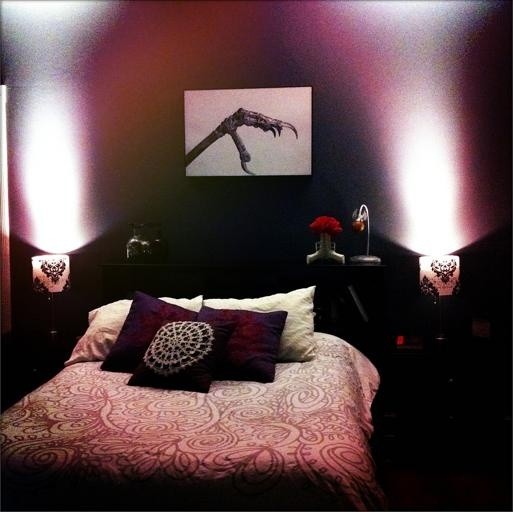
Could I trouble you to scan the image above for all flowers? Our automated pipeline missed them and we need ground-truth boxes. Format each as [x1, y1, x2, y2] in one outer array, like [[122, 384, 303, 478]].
[[308, 216, 341, 240]]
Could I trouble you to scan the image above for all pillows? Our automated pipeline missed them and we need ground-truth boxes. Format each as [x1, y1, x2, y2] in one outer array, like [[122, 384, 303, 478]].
[[64, 296, 202, 366], [191, 306, 287, 384], [0, 264, 384, 512], [126, 318, 230, 393], [202, 285, 318, 362], [100, 289, 199, 374]]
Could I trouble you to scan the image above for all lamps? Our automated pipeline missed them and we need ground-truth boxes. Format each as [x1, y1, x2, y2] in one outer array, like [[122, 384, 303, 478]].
[[417, 255, 460, 338], [29, 254, 71, 379]]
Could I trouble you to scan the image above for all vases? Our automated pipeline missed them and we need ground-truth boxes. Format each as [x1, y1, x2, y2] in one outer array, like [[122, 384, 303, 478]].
[[305, 233, 345, 265]]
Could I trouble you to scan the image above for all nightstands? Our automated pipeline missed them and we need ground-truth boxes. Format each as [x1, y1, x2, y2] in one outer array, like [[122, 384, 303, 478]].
[[377, 336, 422, 444]]
